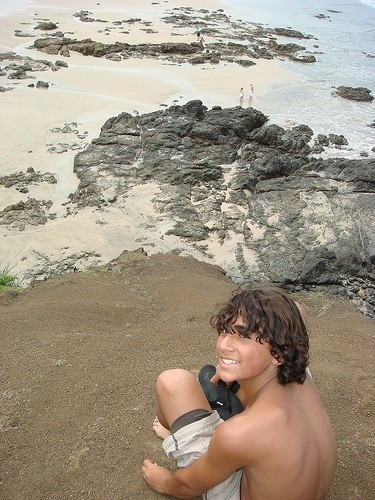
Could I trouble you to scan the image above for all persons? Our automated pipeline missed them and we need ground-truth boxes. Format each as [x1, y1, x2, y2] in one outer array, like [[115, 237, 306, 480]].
[[239, 88, 244, 107], [142, 289, 339, 500], [249, 83, 254, 101]]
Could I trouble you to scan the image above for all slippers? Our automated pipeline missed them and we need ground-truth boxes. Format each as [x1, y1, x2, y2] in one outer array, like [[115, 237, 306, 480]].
[[198, 364, 243, 421]]
